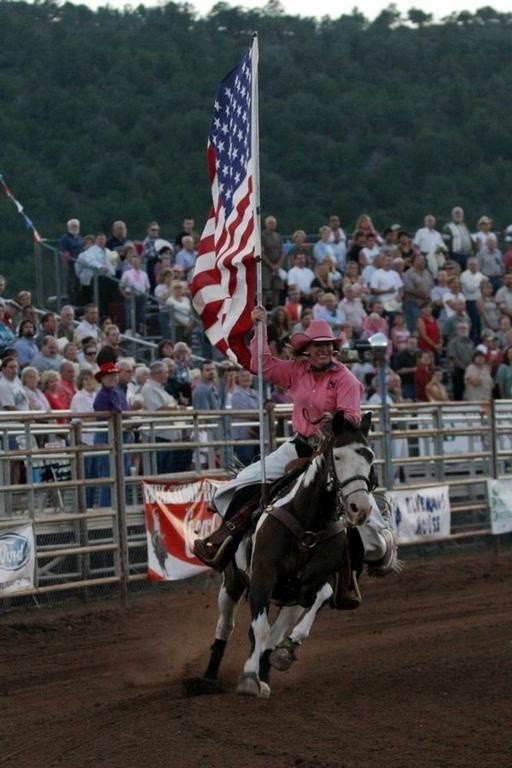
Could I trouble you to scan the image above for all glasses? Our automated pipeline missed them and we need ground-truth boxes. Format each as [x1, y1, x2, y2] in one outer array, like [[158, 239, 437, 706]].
[[86, 351, 100, 357]]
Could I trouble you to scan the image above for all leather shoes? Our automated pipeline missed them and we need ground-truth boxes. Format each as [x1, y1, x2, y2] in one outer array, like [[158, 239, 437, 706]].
[[336, 586, 362, 604], [195, 539, 222, 561]]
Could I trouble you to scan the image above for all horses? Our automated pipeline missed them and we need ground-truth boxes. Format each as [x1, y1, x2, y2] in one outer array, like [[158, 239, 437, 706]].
[[193, 409, 377, 700]]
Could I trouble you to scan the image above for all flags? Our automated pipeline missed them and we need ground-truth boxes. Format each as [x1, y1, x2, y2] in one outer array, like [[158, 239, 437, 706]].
[[188, 42, 253, 377]]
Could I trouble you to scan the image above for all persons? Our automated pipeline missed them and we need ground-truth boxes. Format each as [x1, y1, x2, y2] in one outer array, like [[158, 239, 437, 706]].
[[397, 231, 414, 255], [351, 279, 369, 297], [97, 313, 112, 334], [0, 349, 21, 361], [364, 302, 389, 342], [229, 367, 261, 462], [340, 322, 359, 348], [416, 304, 444, 357], [167, 284, 197, 336], [371, 253, 406, 312], [287, 251, 320, 296], [133, 367, 148, 404], [354, 214, 384, 245], [18, 290, 32, 307], [361, 321, 363, 322], [261, 215, 285, 307], [465, 351, 496, 400], [0, 304, 21, 346], [36, 313, 58, 348], [265, 307, 291, 355], [341, 262, 376, 292], [314, 290, 327, 318], [89, 232, 116, 321], [460, 257, 498, 342], [17, 307, 41, 337], [445, 299, 473, 338], [311, 263, 333, 293], [223, 365, 236, 412], [138, 361, 179, 474], [125, 362, 144, 476], [118, 256, 148, 334], [310, 286, 326, 299], [171, 341, 194, 387], [41, 370, 68, 424], [323, 255, 343, 288], [171, 265, 190, 280], [471, 216, 498, 244], [92, 361, 132, 508], [118, 243, 147, 271], [154, 269, 173, 343], [496, 347, 512, 402], [312, 226, 343, 266], [13, 320, 40, 367], [413, 215, 448, 257], [160, 357, 176, 382], [290, 315, 314, 336], [192, 361, 222, 411], [30, 335, 64, 371], [425, 371, 455, 402], [394, 337, 429, 394], [476, 282, 502, 340], [494, 273, 512, 313], [446, 279, 470, 313], [74, 234, 97, 312], [369, 378, 396, 407], [359, 233, 387, 280], [63, 341, 82, 367], [326, 215, 346, 245], [401, 255, 437, 330], [192, 321, 364, 567], [157, 341, 175, 361], [287, 230, 312, 261], [355, 358, 376, 380], [1, 360, 24, 484], [174, 217, 203, 247], [442, 261, 458, 283], [375, 355, 399, 380], [142, 221, 163, 242], [504, 240, 512, 271], [175, 236, 201, 266], [336, 287, 367, 328], [72, 303, 101, 344], [380, 227, 400, 254], [319, 293, 350, 342], [5, 300, 19, 315], [106, 222, 139, 259], [151, 245, 173, 268], [21, 365, 50, 411], [345, 233, 366, 260], [271, 379, 292, 406], [305, 306, 312, 313], [391, 313, 418, 351], [386, 374, 415, 404], [94, 323, 126, 364], [285, 285, 303, 319], [57, 361, 77, 421], [80, 345, 101, 375], [57, 305, 76, 340], [432, 270, 457, 307], [445, 322, 476, 369], [495, 316, 510, 344], [443, 208, 474, 251], [68, 369, 98, 508], [414, 352, 439, 404], [57, 217, 85, 307], [476, 238, 506, 284], [189, 417, 212, 471], [0, 277, 7, 296], [505, 331, 512, 344], [394, 258, 414, 292], [476, 329, 504, 360], [391, 225, 403, 233]]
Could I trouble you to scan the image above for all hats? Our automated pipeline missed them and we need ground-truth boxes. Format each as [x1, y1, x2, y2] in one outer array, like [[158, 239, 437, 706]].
[[93, 363, 124, 383], [290, 320, 346, 351], [105, 247, 119, 268], [480, 328, 496, 341], [475, 215, 494, 230], [369, 332, 388, 347]]
